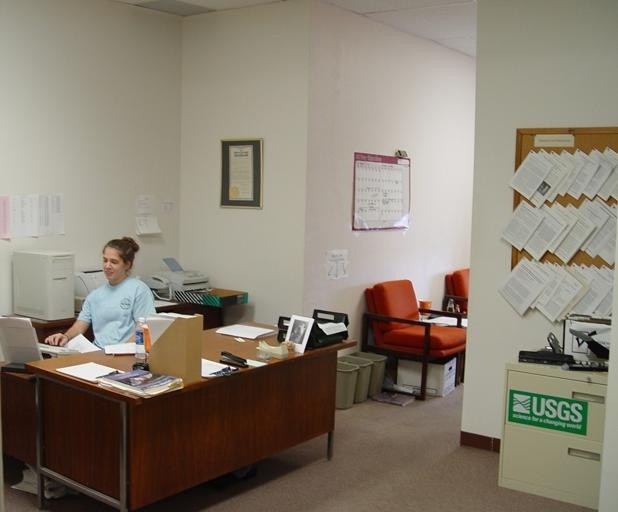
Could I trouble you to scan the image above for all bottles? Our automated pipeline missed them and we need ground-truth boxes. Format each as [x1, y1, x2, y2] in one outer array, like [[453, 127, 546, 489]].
[[447, 298, 454, 312], [135, 317, 145, 359]]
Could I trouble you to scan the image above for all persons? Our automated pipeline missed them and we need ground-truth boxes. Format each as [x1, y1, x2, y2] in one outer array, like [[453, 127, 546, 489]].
[[44, 236, 158, 351]]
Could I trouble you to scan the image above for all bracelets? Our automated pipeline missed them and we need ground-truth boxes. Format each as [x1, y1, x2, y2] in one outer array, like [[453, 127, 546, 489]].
[[65, 334, 69, 341]]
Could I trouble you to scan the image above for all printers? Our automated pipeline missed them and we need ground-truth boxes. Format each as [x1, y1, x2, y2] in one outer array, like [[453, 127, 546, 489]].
[[157, 258, 209, 298]]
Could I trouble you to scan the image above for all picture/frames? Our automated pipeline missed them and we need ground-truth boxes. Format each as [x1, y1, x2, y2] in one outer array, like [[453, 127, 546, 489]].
[[218, 137, 263, 209]]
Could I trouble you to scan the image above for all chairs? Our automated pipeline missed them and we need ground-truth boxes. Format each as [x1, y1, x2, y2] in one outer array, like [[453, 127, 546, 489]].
[[442, 267, 470, 313], [361, 280, 466, 400]]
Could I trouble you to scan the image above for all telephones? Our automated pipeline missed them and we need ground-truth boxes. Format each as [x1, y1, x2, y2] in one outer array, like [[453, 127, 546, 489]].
[[141, 275, 170, 289]]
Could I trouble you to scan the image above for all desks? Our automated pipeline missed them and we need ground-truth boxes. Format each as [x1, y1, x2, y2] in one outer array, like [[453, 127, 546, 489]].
[[0, 300, 224, 360], [419, 308, 467, 327], [0, 323, 358, 512]]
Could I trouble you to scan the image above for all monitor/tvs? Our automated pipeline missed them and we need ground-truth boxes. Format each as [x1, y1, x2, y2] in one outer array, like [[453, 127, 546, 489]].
[[0, 317, 43, 366]]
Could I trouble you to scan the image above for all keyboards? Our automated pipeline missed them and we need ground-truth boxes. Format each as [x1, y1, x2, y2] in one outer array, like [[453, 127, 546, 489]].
[[39, 344, 78, 358]]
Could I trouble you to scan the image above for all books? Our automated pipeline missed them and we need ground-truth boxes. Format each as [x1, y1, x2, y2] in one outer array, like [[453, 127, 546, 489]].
[[96, 369, 184, 399]]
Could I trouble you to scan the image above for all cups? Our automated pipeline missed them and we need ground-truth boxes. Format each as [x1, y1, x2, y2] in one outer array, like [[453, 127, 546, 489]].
[[418, 300, 432, 315]]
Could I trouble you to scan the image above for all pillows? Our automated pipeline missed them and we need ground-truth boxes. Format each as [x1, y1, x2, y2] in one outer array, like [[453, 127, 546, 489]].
[[452, 269, 470, 297], [373, 279, 419, 320]]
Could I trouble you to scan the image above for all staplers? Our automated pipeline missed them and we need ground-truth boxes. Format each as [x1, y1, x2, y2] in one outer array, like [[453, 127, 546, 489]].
[[220, 352, 249, 368]]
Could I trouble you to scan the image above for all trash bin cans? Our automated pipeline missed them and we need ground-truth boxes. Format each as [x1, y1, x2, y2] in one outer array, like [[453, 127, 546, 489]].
[[336, 352, 388, 410]]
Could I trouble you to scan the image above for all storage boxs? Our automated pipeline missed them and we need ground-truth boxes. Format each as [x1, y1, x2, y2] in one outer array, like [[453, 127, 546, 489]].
[[397, 356, 456, 397]]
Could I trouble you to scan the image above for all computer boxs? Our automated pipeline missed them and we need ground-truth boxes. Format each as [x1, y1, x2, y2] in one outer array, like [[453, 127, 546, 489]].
[[13, 250, 76, 321]]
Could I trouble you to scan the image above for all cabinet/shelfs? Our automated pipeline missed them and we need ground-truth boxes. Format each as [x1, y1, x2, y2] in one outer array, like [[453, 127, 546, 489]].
[[497, 362, 608, 510]]
